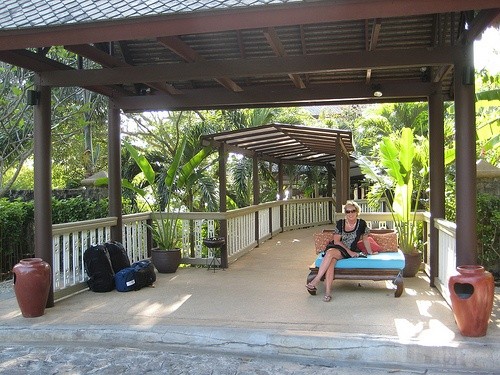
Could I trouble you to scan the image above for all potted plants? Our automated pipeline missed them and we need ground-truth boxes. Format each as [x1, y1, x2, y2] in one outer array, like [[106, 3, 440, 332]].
[[355, 126, 455, 276], [94, 135, 215, 273]]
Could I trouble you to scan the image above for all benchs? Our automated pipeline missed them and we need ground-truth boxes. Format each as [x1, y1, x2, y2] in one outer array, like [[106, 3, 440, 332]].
[[306, 230, 406, 298]]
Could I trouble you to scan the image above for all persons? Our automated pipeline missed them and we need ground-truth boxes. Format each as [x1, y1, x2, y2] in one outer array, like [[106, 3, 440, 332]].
[[306, 201, 378, 302]]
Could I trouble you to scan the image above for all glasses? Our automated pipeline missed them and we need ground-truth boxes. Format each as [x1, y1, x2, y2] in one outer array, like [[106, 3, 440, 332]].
[[345, 209, 356, 213]]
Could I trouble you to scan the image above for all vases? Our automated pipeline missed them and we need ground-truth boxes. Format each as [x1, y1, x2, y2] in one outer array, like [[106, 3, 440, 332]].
[[12, 258, 52, 318], [448, 263, 494, 338]]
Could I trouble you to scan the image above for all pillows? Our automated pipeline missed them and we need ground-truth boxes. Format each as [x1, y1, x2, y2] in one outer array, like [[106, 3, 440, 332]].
[[357, 237, 382, 254], [312, 232, 333, 255], [367, 231, 398, 253]]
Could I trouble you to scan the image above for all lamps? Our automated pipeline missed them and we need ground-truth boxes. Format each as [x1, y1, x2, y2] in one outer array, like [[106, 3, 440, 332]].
[[25, 89, 37, 105], [137, 84, 151, 95], [420, 66, 430, 82], [372, 84, 383, 97], [462, 66, 475, 85]]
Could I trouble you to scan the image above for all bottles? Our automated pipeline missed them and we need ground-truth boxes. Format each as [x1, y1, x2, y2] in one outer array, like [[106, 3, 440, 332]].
[[12, 258, 52, 318], [448, 265, 495, 337]]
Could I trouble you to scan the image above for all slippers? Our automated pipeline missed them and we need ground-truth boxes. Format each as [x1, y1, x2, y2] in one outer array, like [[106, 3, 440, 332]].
[[305, 283, 317, 295], [322, 296, 331, 302]]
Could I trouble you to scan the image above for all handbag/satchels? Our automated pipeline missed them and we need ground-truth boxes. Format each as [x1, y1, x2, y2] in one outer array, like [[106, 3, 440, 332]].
[[115, 260, 157, 292], [105, 240, 130, 273], [83, 244, 115, 292]]
